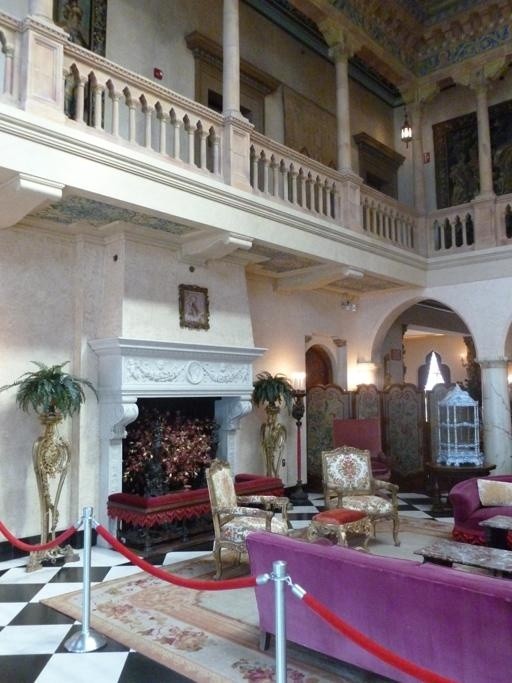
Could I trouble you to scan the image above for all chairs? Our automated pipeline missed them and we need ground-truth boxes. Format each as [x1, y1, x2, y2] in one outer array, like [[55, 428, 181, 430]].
[[315, 444, 408, 550], [330, 415, 394, 484], [203, 454, 297, 583]]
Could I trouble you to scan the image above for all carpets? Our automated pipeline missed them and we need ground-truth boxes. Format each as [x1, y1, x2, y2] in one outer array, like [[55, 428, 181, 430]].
[[39, 511, 512, 682]]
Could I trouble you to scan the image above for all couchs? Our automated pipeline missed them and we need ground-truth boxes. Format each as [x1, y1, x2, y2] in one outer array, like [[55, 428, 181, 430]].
[[447, 473, 512, 549], [244, 530, 512, 682]]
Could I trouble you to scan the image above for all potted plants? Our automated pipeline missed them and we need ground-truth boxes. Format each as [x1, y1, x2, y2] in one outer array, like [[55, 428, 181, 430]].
[[248, 366, 298, 418], [0, 359, 103, 422]]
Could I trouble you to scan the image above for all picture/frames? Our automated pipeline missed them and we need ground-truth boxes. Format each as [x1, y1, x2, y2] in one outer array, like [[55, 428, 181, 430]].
[[178, 279, 212, 330]]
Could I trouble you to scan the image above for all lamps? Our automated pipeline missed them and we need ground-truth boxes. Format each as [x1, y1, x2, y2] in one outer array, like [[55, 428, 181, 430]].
[[290, 386, 311, 507], [339, 289, 361, 312], [402, 106, 414, 149]]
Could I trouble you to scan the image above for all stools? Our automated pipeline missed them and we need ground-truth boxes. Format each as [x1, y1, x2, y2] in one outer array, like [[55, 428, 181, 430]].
[[307, 505, 377, 549]]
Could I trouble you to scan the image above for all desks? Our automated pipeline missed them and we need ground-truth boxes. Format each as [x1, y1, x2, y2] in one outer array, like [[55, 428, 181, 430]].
[[426, 459, 499, 515]]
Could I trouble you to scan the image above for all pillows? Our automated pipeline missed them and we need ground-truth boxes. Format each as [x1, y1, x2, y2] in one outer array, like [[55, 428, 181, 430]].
[[476, 477, 512, 507]]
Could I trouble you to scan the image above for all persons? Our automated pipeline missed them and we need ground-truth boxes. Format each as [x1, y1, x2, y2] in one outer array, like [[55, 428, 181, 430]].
[[446, 145, 479, 232], [60, 0, 91, 51]]
